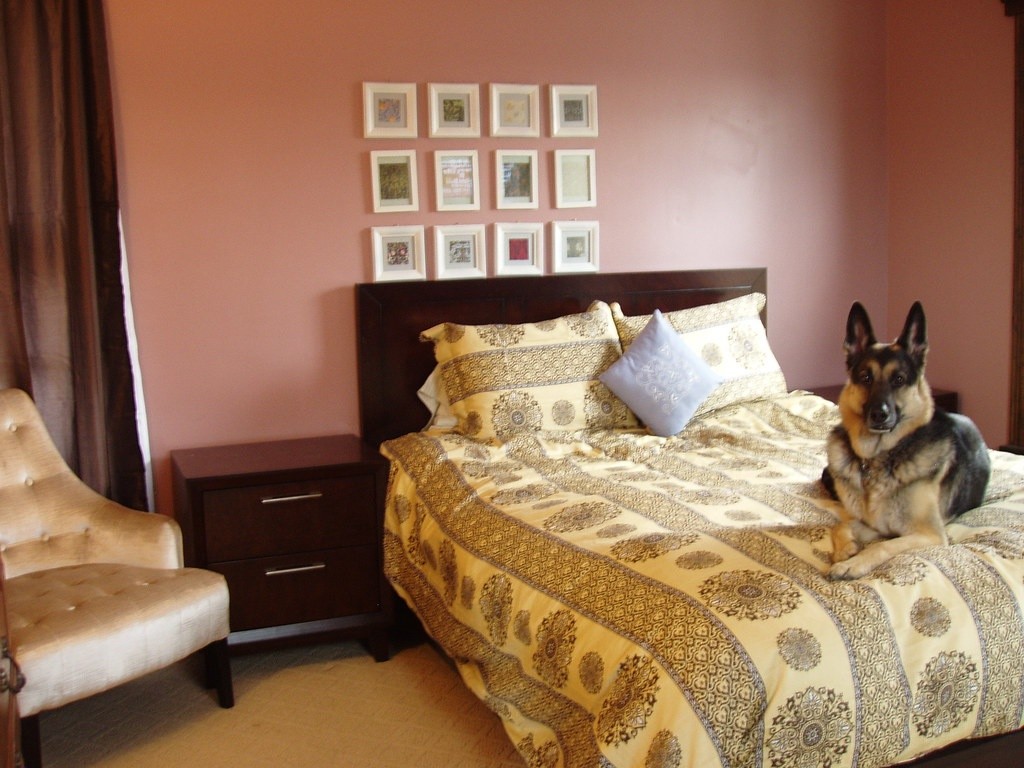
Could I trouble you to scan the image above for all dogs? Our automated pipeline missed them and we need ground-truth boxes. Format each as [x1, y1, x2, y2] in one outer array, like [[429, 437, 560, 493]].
[[820, 297, 991, 584]]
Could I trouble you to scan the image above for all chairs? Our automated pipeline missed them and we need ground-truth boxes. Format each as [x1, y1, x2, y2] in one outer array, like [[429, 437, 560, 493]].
[[0, 385, 242, 768]]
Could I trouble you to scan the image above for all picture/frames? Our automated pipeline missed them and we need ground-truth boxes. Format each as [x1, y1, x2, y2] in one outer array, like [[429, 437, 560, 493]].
[[362, 79, 422, 140], [369, 224, 428, 282], [489, 81, 543, 140], [552, 147, 599, 210], [425, 81, 483, 140], [493, 148, 542, 211], [431, 147, 482, 214], [549, 81, 601, 141], [491, 219, 547, 277], [550, 217, 602, 276], [430, 222, 489, 279], [369, 147, 421, 217]]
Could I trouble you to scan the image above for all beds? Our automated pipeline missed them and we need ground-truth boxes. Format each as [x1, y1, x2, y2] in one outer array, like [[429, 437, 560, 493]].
[[350, 263, 1024, 768]]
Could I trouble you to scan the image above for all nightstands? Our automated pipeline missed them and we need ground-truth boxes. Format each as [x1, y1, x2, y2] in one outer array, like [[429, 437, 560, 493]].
[[166, 431, 396, 693], [789, 384, 960, 420]]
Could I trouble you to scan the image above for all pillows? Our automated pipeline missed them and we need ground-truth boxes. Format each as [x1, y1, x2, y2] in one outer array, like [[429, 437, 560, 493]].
[[417, 300, 637, 440], [595, 306, 721, 436], [611, 291, 790, 435]]
